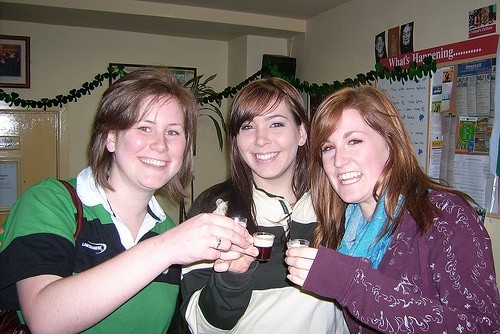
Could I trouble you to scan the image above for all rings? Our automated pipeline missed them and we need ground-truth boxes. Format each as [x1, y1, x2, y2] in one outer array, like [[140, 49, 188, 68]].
[[215, 239, 223, 250]]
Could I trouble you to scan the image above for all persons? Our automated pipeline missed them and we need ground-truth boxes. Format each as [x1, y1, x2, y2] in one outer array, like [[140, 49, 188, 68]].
[[285, 87, 500, 334], [180, 76, 351, 334], [376, 36, 386, 61], [400, 24, 413, 55], [1, 67, 264, 334]]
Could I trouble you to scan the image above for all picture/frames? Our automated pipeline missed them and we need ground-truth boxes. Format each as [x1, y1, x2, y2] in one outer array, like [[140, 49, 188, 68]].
[[0, 35, 31, 89], [108, 62, 197, 89]]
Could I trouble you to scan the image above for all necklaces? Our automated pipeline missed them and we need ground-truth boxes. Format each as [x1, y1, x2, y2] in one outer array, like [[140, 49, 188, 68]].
[[290, 203, 295, 207]]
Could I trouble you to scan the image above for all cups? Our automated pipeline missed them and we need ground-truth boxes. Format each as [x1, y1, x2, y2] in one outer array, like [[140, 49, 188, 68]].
[[252, 232, 275, 261], [231, 217, 247, 229], [286, 239, 310, 248]]
